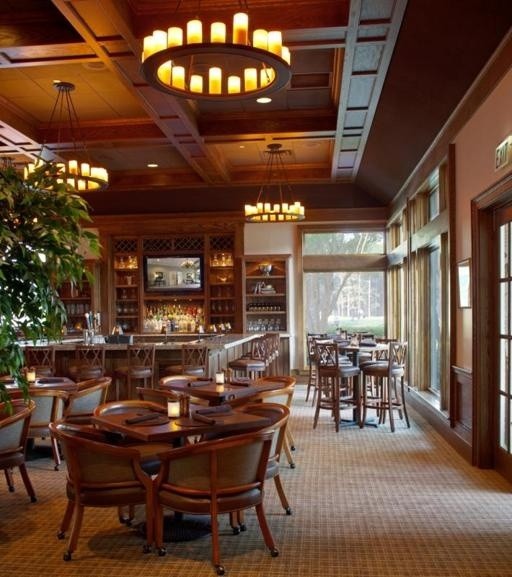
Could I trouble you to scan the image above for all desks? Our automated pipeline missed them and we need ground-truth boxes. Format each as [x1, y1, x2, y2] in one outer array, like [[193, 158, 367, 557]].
[[91, 406, 271, 543], [161, 379, 266, 407], [326, 343, 389, 429]]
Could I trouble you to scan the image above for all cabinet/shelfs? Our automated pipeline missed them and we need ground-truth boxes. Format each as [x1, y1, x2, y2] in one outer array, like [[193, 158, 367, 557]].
[[50, 259, 100, 335], [241, 255, 290, 338], [113, 250, 240, 335]]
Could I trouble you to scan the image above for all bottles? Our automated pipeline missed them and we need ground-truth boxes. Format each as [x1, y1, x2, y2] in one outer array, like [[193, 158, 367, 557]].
[[117, 303, 138, 315], [121, 288, 127, 299], [145, 303, 203, 334], [249, 302, 281, 311], [130, 289, 136, 299], [114, 320, 128, 335]]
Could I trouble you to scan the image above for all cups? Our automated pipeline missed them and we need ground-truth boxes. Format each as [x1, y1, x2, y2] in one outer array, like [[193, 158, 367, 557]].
[[82, 330, 94, 347]]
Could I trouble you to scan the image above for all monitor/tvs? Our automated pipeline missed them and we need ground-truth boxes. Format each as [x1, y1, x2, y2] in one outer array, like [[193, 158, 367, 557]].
[[143, 254, 204, 295]]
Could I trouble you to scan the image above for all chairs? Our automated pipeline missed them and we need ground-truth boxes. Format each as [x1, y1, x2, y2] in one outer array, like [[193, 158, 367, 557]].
[[313, 341, 362, 433], [359, 334, 375, 343], [375, 338, 403, 420], [63, 376, 112, 431], [159, 376, 211, 417], [311, 337, 335, 408], [164, 344, 207, 381], [152, 430, 279, 576], [113, 344, 155, 401], [266, 332, 279, 376], [305, 333, 328, 402], [238, 375, 296, 469], [93, 398, 169, 524], [24, 345, 57, 377], [359, 341, 410, 433], [8, 389, 69, 471], [228, 339, 266, 381], [234, 403, 292, 533], [0, 398, 38, 502], [263, 334, 275, 376], [47, 421, 167, 561], [242, 338, 269, 378], [257, 336, 272, 376], [67, 344, 106, 383]]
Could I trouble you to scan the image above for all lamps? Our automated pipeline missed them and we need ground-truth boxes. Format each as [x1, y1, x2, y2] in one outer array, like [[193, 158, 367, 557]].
[[245, 144, 305, 223], [23, 82, 108, 194], [141, 3, 292, 104]]
